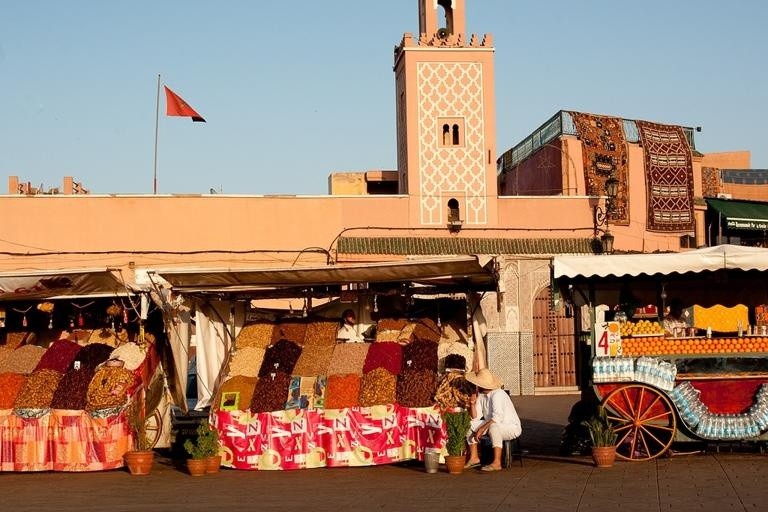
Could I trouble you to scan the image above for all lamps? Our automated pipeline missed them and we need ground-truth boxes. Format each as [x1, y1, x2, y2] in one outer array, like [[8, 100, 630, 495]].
[[450, 219, 463, 233], [594, 174, 619, 255]]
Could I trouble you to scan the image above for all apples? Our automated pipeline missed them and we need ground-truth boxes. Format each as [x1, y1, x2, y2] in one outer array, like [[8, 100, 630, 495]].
[[620, 319, 665, 337]]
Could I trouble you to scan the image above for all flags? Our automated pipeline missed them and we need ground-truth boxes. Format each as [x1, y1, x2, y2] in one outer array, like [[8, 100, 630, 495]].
[[163, 84, 207, 124]]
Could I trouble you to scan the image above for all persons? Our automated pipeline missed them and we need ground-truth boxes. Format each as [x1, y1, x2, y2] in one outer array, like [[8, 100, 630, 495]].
[[664, 303, 698, 337], [464, 368, 522, 472], [337, 309, 377, 343]]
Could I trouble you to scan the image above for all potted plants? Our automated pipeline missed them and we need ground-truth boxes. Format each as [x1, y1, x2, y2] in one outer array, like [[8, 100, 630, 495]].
[[123, 402, 154, 474], [582, 409, 617, 468], [186, 430, 208, 477], [444, 410, 472, 474], [198, 420, 221, 473]]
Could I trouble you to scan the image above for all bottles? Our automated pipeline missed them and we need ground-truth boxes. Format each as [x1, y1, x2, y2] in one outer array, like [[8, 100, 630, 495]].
[[737, 326, 743, 337], [754, 325, 758, 335], [689, 327, 695, 337], [673, 327, 678, 337], [668, 380, 768, 441], [761, 325, 766, 335], [634, 355, 678, 392], [681, 326, 686, 337], [706, 326, 712, 339], [747, 325, 752, 335], [592, 354, 635, 383]]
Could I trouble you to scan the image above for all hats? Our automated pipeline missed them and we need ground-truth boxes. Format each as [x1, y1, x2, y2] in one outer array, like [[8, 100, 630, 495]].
[[464, 368, 500, 389]]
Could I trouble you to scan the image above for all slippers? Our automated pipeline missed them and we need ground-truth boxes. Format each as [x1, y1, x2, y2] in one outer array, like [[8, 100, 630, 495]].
[[481, 464, 498, 472], [464, 460, 481, 469]]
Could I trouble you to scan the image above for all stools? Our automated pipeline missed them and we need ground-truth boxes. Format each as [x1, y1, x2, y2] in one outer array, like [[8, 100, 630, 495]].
[[504, 440, 525, 469]]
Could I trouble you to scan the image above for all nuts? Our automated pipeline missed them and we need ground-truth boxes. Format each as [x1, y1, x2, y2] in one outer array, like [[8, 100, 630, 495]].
[[212, 316, 474, 414], [0, 327, 156, 412]]
